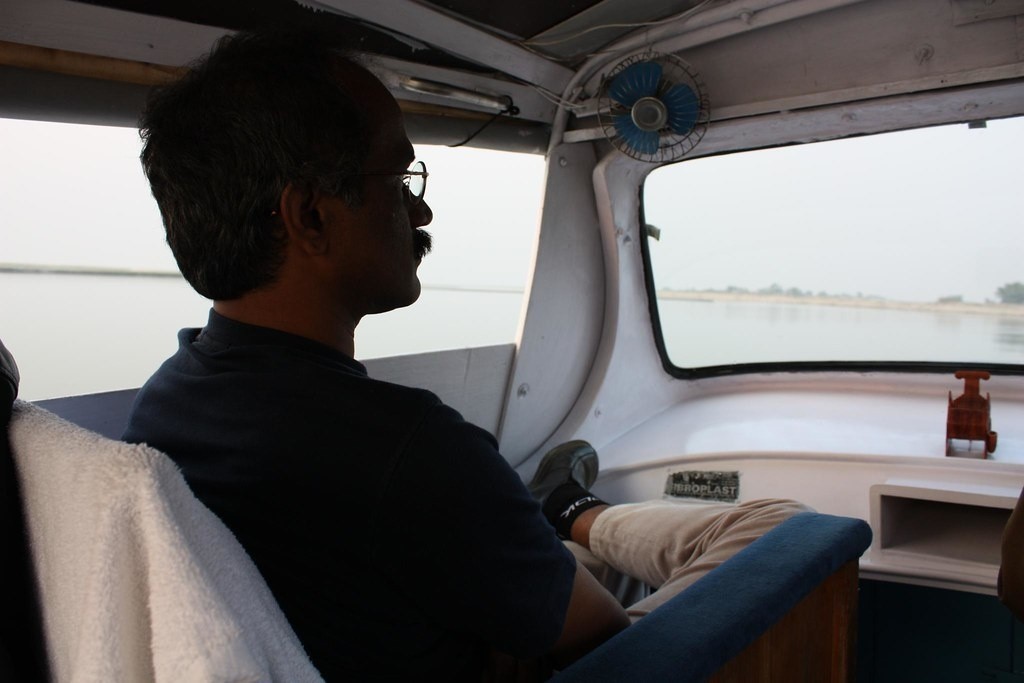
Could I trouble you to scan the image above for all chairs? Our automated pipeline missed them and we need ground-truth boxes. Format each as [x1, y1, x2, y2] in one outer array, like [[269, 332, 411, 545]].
[[6, 398, 874, 683]]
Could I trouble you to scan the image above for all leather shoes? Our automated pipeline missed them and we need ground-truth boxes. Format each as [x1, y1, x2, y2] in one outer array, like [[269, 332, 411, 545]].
[[527, 440, 598, 509]]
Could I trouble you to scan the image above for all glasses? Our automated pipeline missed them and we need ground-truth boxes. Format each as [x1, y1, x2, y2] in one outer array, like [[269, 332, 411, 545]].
[[270, 161, 428, 217]]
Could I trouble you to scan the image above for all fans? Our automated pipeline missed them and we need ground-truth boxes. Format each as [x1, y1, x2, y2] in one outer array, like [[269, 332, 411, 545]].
[[596, 48, 713, 165]]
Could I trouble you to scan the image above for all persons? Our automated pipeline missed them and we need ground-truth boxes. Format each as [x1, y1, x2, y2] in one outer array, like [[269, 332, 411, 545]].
[[122, 26, 817, 683]]
